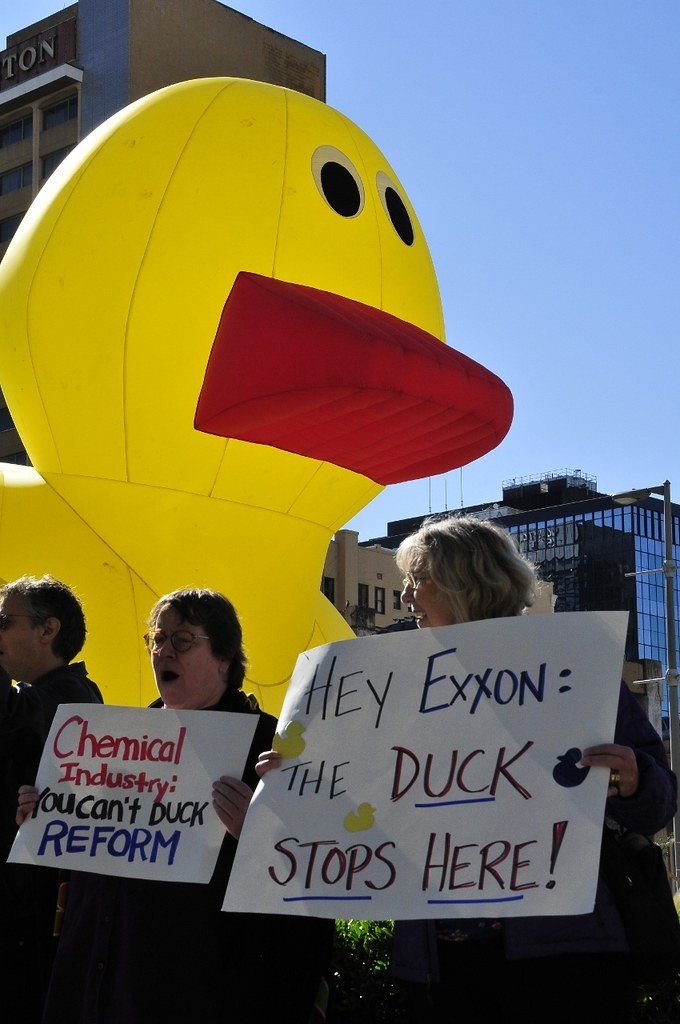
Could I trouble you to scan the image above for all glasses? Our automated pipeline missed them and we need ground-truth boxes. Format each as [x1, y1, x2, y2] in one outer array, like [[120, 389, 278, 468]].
[[143, 631, 211, 653], [0, 613, 40, 629], [403, 571, 433, 589]]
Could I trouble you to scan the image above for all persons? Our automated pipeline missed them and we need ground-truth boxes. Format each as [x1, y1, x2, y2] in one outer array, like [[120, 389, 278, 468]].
[[255, 518, 680, 1024], [0, 577, 104, 1024], [15, 589, 337, 1024]]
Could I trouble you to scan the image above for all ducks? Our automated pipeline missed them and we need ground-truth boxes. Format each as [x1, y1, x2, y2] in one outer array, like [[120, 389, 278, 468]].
[[0, 75, 518, 720]]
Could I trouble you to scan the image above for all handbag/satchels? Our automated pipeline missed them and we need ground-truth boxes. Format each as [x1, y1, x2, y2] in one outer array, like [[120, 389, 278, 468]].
[[609, 828, 680, 984]]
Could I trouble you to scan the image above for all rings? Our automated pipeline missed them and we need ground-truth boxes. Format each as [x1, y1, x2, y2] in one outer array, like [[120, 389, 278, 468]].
[[611, 769, 619, 786]]
